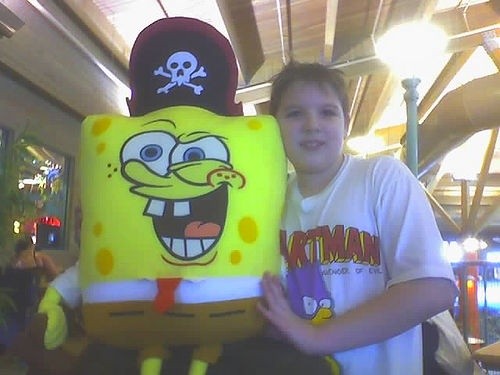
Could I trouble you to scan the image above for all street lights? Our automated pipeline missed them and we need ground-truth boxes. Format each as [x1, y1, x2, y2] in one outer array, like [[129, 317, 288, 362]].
[[373, 21, 451, 177]]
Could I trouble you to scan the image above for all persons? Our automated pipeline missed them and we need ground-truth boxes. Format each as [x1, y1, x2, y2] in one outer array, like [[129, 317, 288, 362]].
[[255, 54, 457, 374], [9, 237, 61, 294]]
